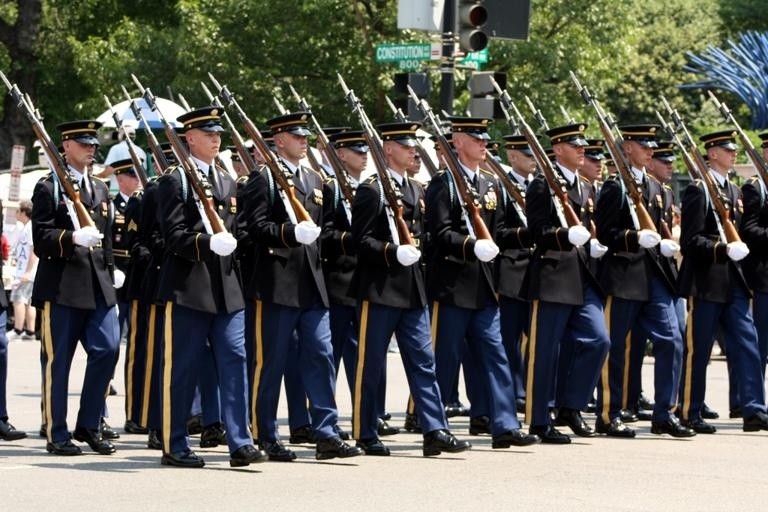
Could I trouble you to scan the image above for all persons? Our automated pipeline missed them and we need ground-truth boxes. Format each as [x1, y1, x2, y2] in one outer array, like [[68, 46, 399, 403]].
[[103, 125, 147, 171], [156, 106, 269, 468], [351, 123, 471, 457], [741, 132, 768, 379], [108, 158, 144, 271], [5, 202, 39, 339], [676, 130, 768, 433], [0, 280, 27, 441], [524, 123, 595, 444], [424, 116, 541, 449], [30, 119, 121, 457], [579, 139, 607, 202], [593, 125, 696, 438], [324, 131, 400, 436], [245, 112, 362, 460], [646, 142, 691, 332], [494, 134, 542, 413]]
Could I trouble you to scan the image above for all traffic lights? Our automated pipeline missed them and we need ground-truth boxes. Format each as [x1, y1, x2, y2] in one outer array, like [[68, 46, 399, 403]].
[[473, 70, 507, 121], [395, 71, 429, 121], [456, 1, 491, 52]]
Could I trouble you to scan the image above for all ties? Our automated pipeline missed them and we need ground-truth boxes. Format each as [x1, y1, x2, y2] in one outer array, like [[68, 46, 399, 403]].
[[642, 175, 649, 195], [573, 176, 580, 198], [402, 178, 413, 205], [209, 166, 221, 198], [723, 180, 731, 199], [473, 174, 479, 195], [295, 169, 301, 181], [82, 178, 91, 204]]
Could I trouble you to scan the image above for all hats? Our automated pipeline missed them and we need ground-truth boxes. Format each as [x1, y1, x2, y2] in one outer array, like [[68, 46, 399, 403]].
[[758, 133, 768, 148], [699, 130, 739, 150], [226, 146, 254, 160], [503, 134, 541, 156], [57, 121, 102, 145], [174, 128, 187, 141], [546, 123, 589, 146], [415, 136, 425, 157], [448, 116, 493, 139], [313, 127, 350, 141], [177, 107, 224, 132], [545, 149, 557, 161], [266, 113, 312, 135], [700, 154, 711, 166], [618, 125, 658, 147], [486, 141, 501, 161], [604, 153, 617, 165], [429, 133, 455, 150], [260, 131, 276, 151], [652, 142, 677, 162], [110, 158, 144, 174], [585, 139, 606, 160], [145, 142, 175, 160], [377, 122, 422, 147], [330, 131, 370, 153]]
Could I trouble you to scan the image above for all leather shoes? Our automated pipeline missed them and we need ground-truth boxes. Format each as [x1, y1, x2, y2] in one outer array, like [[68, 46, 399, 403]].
[[702, 406, 718, 419], [730, 410, 743, 418], [40, 428, 72, 439], [378, 418, 400, 435], [315, 437, 364, 460], [101, 418, 120, 439], [290, 425, 316, 443], [687, 418, 715, 434], [652, 418, 696, 437], [569, 410, 597, 437], [596, 416, 635, 438], [469, 417, 522, 435], [259, 441, 296, 461], [73, 431, 116, 454], [356, 438, 390, 456], [637, 412, 652, 420], [148, 431, 162, 448], [161, 449, 204, 468], [200, 425, 228, 448], [230, 445, 268, 466], [492, 429, 541, 448], [530, 425, 571, 444], [405, 415, 417, 430], [619, 411, 638, 422], [125, 422, 148, 433], [743, 411, 768, 432], [424, 429, 471, 456], [447, 403, 471, 415], [0, 418, 27, 440], [46, 439, 82, 455], [640, 397, 654, 410]]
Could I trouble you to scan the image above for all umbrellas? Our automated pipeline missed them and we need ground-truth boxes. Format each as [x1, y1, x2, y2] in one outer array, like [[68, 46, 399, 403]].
[[96, 97, 188, 128]]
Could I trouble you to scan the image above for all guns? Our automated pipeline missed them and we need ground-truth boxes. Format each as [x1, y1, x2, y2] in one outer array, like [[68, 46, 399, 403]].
[[200, 80, 256, 172], [384, 94, 437, 177], [570, 70, 657, 232], [661, 96, 742, 243], [0, 70, 97, 229], [208, 72, 313, 224], [708, 90, 767, 189], [289, 85, 355, 206], [131, 73, 228, 234], [407, 84, 493, 240], [489, 75, 580, 227], [483, 148, 525, 209], [337, 73, 413, 245], [122, 85, 170, 175], [654, 110, 701, 183], [104, 94, 149, 187]]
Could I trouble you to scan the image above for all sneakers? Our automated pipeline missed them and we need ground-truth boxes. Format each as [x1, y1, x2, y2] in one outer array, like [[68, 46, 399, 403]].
[[6, 330, 23, 342], [22, 332, 36, 341]]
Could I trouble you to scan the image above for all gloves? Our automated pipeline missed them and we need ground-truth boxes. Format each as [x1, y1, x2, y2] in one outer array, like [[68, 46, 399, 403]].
[[114, 269, 125, 289], [474, 239, 499, 261], [728, 241, 750, 261], [396, 244, 421, 265], [568, 225, 590, 245], [295, 220, 321, 244], [661, 238, 680, 256], [210, 232, 237, 256], [75, 227, 104, 248], [590, 238, 609, 258], [638, 229, 661, 249]]
